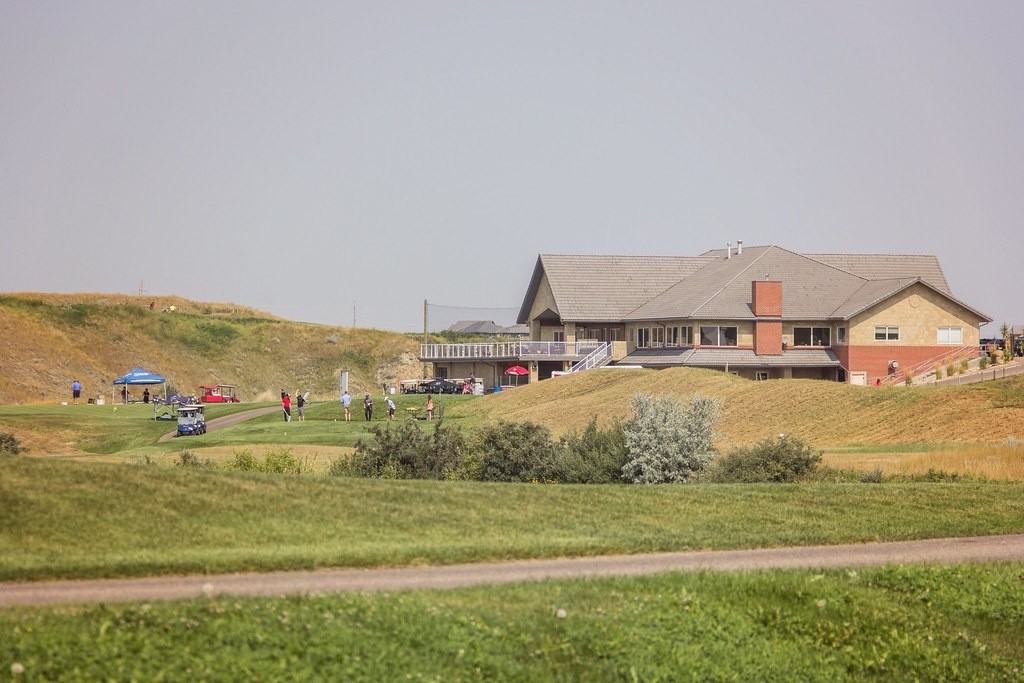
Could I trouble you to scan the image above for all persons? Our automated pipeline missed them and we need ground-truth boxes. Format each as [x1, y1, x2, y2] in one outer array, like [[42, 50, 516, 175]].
[[281, 389, 286, 398], [282, 394, 292, 422], [874, 379, 880, 386], [72, 381, 80, 404], [425, 395, 434, 421], [184, 411, 200, 418], [462, 381, 470, 395], [383, 396, 396, 422], [120, 386, 129, 404], [143, 388, 148, 403], [986, 341, 990, 351], [150, 302, 155, 308], [340, 391, 351, 421], [363, 393, 373, 421], [470, 373, 476, 394], [295, 389, 306, 421]]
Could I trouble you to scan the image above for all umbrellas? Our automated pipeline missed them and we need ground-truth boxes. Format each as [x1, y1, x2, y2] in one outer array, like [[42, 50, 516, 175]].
[[505, 366, 530, 388], [419, 379, 460, 394]]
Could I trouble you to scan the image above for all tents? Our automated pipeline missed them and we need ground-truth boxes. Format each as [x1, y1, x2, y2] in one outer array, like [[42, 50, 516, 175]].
[[113, 367, 166, 405], [152, 392, 198, 421]]
